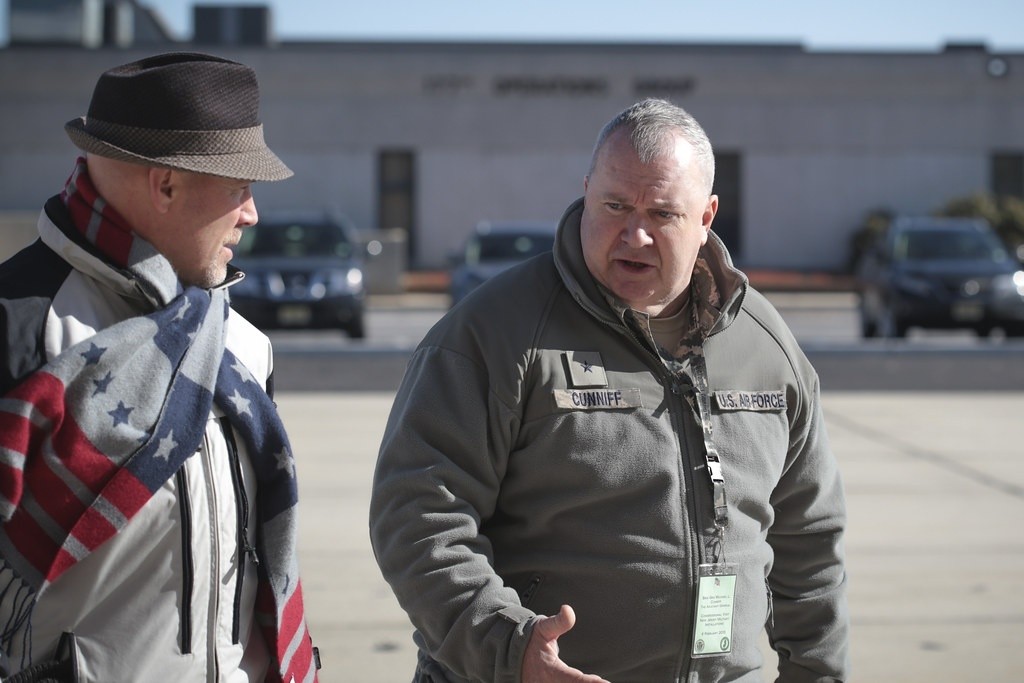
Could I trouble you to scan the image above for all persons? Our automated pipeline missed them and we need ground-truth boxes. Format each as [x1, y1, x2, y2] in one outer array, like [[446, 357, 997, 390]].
[[0, 53, 323, 683], [368, 96, 850, 683]]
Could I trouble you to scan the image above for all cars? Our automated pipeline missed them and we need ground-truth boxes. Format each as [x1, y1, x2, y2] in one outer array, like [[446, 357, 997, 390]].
[[443, 221, 553, 308], [853, 212, 1023, 340], [224, 210, 364, 343]]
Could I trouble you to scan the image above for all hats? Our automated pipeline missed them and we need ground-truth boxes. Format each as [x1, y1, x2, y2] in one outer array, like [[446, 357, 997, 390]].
[[64, 52, 294, 182]]
